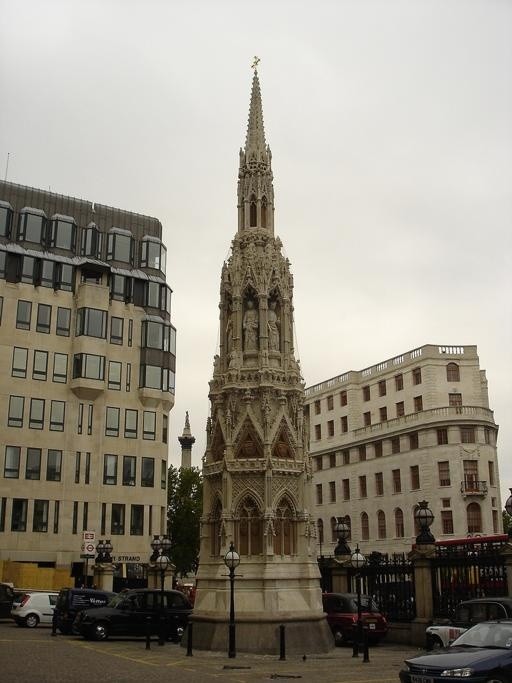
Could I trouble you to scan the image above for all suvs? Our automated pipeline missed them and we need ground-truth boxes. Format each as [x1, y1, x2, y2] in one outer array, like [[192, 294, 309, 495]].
[[1, 582, 216, 683]]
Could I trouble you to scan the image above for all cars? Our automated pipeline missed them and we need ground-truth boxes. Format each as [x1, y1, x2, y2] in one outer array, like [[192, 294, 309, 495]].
[[322, 593, 389, 646], [425, 596, 511, 651], [395, 618, 512, 682]]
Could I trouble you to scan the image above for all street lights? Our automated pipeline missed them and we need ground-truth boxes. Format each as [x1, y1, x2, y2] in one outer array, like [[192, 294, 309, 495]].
[[414, 497, 435, 544], [150, 534, 174, 647], [351, 541, 365, 652], [333, 517, 351, 554], [95, 539, 114, 563], [503, 486, 511, 537], [220, 538, 244, 659]]
